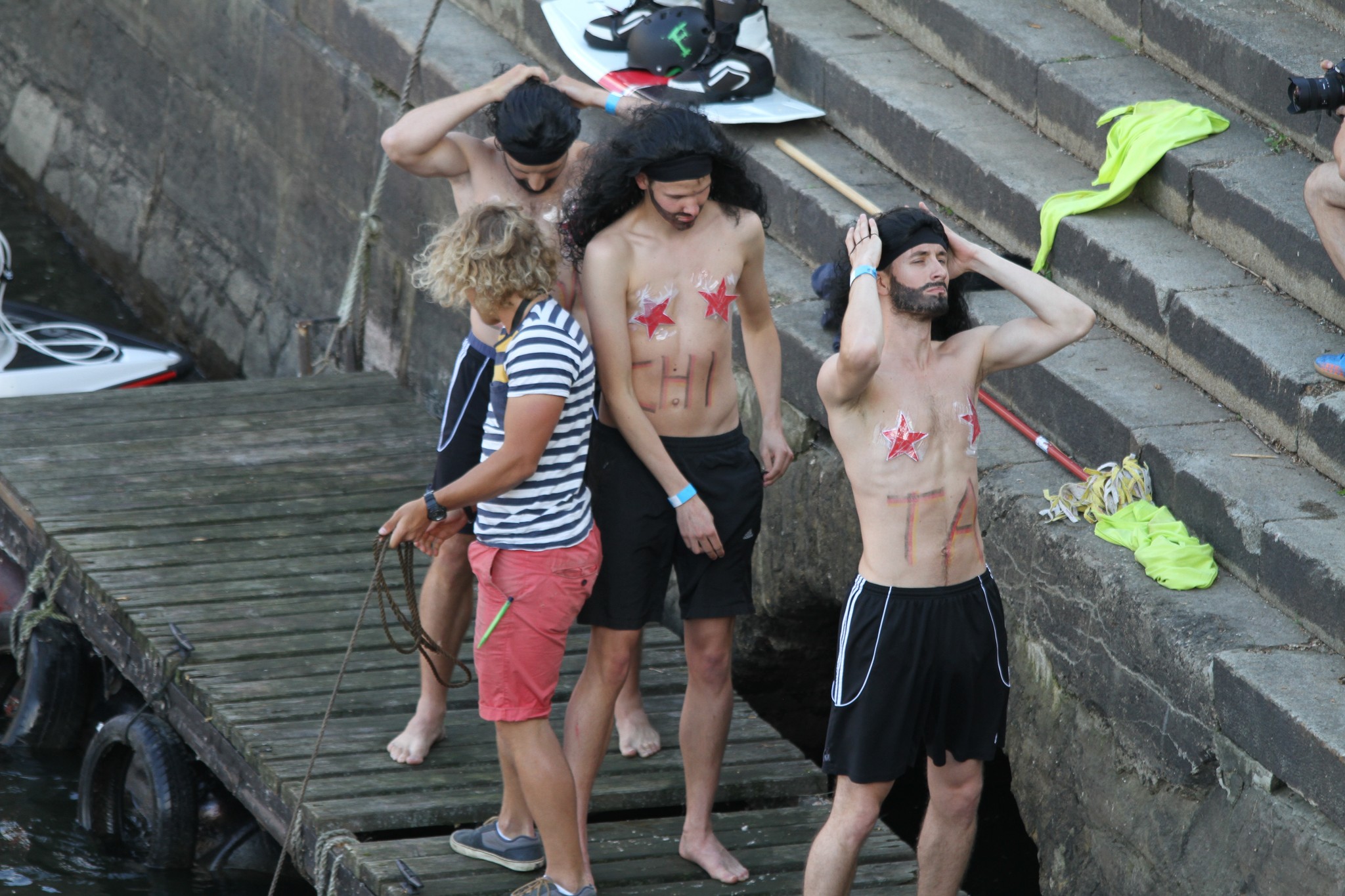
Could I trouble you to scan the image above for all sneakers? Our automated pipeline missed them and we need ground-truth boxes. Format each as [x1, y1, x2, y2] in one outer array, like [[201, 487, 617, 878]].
[[509, 876, 594, 896], [449, 816, 544, 871]]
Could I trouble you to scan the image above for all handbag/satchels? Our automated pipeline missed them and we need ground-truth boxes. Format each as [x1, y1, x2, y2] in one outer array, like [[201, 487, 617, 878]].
[[585, 0, 668, 51]]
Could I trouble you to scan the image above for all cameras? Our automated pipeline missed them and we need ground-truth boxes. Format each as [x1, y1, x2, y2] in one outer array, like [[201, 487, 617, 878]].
[[1287, 58, 1345, 124]]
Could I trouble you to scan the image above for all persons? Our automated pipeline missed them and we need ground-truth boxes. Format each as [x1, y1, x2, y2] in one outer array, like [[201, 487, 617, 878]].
[[375, 201, 608, 896], [560, 104, 794, 883], [380, 63, 661, 764], [1305, 60, 1345, 384], [798, 208, 1096, 896]]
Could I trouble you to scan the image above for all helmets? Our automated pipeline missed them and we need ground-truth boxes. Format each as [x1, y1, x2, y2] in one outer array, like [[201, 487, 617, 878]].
[[627, 6, 716, 79]]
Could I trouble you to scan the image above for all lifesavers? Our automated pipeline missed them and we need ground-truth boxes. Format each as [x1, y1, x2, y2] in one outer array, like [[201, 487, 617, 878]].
[[1, 608, 102, 753], [81, 710, 202, 882]]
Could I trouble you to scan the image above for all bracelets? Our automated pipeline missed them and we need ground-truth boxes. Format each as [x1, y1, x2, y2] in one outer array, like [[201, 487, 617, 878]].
[[666, 484, 698, 508], [848, 233, 880, 257], [849, 265, 878, 287], [605, 94, 620, 115]]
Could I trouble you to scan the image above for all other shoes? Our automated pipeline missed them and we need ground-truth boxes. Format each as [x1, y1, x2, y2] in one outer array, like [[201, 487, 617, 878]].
[[1312, 353, 1345, 381]]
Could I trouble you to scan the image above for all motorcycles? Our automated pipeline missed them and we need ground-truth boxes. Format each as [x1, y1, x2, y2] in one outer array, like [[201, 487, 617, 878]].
[[0, 295, 192, 396]]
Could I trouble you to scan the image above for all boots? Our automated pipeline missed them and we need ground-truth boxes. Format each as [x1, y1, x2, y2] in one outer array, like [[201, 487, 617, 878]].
[[636, 1, 774, 107]]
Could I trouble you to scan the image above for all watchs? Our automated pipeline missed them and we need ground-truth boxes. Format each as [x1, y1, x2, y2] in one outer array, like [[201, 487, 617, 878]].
[[423, 489, 448, 522]]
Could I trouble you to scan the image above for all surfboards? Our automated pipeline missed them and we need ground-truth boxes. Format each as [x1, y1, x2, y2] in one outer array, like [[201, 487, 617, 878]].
[[537, 0, 828, 123]]
